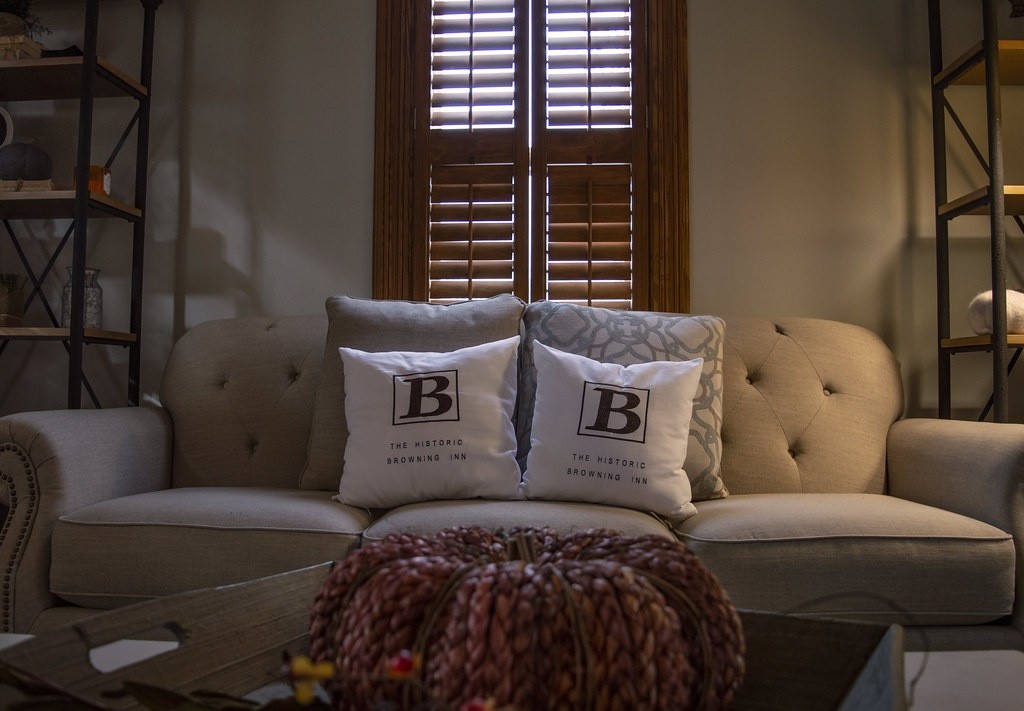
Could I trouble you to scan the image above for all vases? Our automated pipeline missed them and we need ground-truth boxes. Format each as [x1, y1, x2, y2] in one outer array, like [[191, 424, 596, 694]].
[[60, 267, 103, 330]]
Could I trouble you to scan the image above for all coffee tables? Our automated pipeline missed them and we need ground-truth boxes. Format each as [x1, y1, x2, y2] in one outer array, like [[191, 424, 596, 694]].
[[0, 561, 906, 711]]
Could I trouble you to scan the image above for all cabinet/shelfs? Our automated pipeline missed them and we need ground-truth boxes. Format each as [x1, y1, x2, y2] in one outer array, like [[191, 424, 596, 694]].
[[0, 0, 163, 409], [928, 0, 1024, 423]]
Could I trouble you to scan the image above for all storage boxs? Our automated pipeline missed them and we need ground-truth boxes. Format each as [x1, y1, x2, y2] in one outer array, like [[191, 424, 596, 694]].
[[0, 35, 42, 61]]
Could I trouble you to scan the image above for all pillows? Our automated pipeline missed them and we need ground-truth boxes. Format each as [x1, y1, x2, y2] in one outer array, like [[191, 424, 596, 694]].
[[521, 339, 705, 521], [297, 292, 527, 487], [515, 300, 730, 502], [330, 335, 529, 516]]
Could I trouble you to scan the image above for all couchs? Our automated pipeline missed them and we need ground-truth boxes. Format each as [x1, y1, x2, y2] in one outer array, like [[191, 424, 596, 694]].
[[0, 310, 1024, 635]]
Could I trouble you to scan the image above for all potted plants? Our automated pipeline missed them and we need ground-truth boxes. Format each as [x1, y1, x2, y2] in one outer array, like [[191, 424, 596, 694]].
[[0, 0, 53, 40]]
